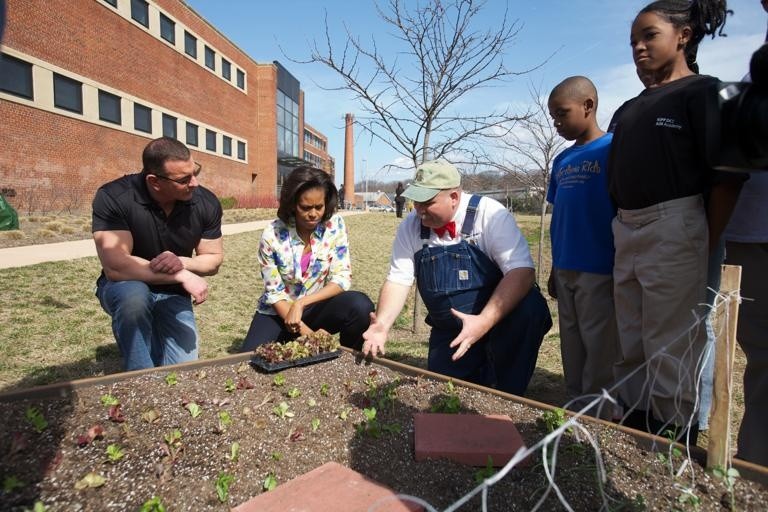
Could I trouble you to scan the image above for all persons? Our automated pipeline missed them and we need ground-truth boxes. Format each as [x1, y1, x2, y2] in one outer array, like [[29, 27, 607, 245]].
[[361, 158, 553, 396], [87, 136, 226, 373], [338, 184, 344, 210], [723, 2, 768, 469], [607, 62, 655, 135], [606, 0, 753, 451], [240, 165, 371, 356], [395, 181, 408, 217], [546, 75, 623, 422]]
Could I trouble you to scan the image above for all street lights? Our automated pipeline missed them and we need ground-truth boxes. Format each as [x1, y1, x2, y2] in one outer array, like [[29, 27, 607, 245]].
[[362, 159, 369, 210]]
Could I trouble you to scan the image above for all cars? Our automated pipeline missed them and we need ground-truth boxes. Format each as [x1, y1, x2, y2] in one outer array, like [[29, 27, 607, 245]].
[[368, 202, 392, 213]]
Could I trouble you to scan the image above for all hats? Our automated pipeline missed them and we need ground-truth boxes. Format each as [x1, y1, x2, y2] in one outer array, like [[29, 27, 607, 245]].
[[399, 162, 462, 203]]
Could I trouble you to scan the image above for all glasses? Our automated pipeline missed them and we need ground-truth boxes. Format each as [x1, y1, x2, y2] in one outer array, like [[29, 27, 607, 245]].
[[156, 162, 202, 184]]
